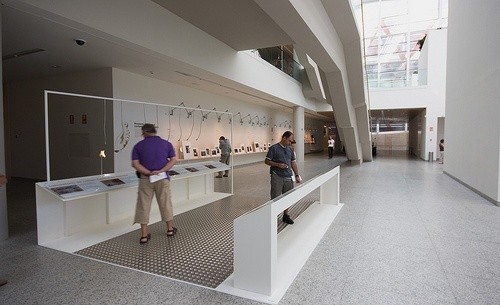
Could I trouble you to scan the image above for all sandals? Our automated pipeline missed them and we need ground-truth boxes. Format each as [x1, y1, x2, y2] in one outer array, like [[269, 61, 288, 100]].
[[166, 226, 177, 237], [140, 234, 151, 245]]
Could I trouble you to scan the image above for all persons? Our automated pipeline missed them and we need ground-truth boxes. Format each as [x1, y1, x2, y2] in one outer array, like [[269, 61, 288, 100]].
[[216, 136, 231, 178], [265, 131, 302, 224], [439, 139, 445, 164], [131, 123, 177, 243], [328, 136, 335, 159]]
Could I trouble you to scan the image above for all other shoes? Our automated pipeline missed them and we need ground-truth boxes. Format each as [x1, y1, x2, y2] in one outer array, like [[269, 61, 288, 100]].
[[282, 215, 294, 224], [216, 174, 228, 178]]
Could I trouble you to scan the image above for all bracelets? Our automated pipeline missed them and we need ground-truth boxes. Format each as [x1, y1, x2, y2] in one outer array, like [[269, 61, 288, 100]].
[[295, 175, 298, 177]]
